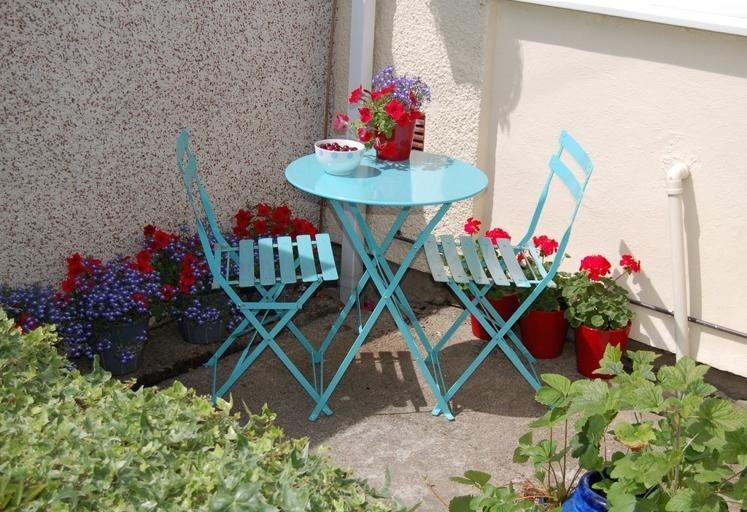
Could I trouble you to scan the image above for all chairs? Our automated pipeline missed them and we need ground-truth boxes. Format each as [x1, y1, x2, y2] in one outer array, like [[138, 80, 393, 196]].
[[419, 126, 594, 417], [168, 127, 333, 421]]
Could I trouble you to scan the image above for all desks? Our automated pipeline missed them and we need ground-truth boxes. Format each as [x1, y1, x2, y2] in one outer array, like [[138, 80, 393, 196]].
[[276, 147, 486, 423]]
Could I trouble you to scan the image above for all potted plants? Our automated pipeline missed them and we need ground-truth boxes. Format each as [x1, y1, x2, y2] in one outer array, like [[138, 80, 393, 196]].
[[537, 337, 747, 512]]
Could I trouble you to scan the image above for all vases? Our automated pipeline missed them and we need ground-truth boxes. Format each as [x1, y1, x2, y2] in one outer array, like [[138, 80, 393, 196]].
[[573, 323, 630, 380], [173, 294, 232, 343], [515, 307, 572, 359], [376, 117, 416, 160], [468, 288, 514, 340], [89, 311, 152, 376]]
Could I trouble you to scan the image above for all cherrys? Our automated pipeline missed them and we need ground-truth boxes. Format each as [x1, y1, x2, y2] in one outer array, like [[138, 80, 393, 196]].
[[319, 142, 358, 151]]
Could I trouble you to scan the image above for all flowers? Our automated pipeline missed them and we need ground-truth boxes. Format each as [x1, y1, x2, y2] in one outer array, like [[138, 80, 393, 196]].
[[336, 66, 433, 134], [1, 277, 85, 360], [507, 232, 567, 311], [136, 223, 248, 328], [234, 202, 321, 294], [566, 251, 643, 325], [455, 214, 519, 295], [59, 247, 158, 359]]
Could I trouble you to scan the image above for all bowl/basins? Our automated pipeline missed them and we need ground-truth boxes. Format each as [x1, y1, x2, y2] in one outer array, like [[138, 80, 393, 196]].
[[314, 138, 365, 175]]
